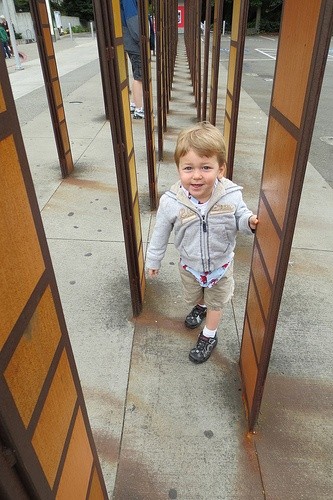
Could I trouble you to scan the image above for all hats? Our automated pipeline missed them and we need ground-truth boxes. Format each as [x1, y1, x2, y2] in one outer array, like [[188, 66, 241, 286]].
[[0, 16, 5, 19]]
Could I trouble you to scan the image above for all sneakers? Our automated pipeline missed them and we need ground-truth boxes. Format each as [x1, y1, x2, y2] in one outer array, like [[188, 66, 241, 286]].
[[130, 105, 144, 113], [185, 304, 207, 328], [133, 109, 155, 119], [189, 331, 218, 364]]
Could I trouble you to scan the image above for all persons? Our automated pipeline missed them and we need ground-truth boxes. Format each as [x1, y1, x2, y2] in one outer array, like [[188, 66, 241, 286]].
[[146, 122, 259, 364], [119, 0, 156, 120], [0, 16, 14, 58]]
